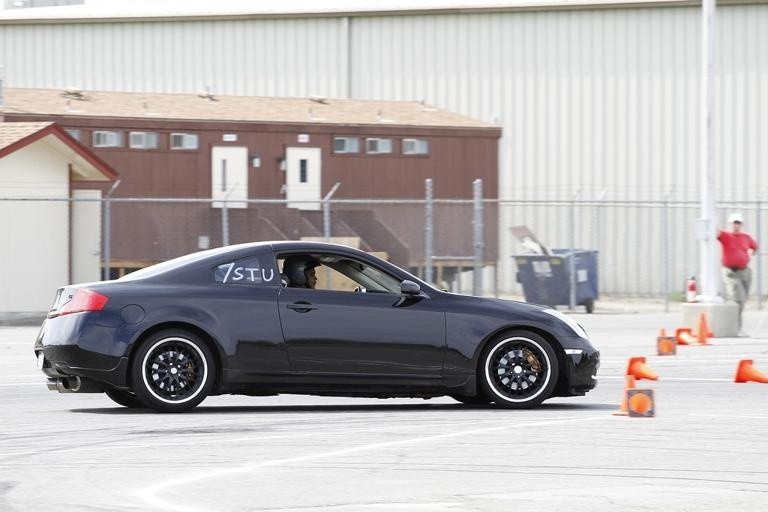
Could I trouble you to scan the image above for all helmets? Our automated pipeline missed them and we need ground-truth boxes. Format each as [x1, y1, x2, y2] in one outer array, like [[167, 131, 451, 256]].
[[283, 255, 321, 285]]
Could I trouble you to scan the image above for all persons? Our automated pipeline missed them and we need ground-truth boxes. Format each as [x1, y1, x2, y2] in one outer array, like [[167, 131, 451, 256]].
[[280, 254, 322, 291], [714, 210, 761, 339]]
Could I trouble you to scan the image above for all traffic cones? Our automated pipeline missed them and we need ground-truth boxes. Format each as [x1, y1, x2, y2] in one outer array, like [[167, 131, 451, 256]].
[[675, 328, 695, 346], [694, 312, 715, 346], [626, 356, 660, 382], [656, 328, 676, 356], [611, 374, 637, 417], [734, 359, 768, 384], [625, 387, 656, 419]]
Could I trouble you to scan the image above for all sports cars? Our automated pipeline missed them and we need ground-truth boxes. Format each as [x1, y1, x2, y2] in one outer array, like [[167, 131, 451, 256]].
[[34, 240, 601, 413]]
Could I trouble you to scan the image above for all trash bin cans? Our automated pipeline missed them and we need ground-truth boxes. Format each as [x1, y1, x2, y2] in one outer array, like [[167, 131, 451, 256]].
[[510, 248, 598, 313]]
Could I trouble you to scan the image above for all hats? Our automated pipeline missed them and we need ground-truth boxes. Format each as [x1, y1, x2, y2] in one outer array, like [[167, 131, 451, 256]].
[[729, 213, 745, 224]]
